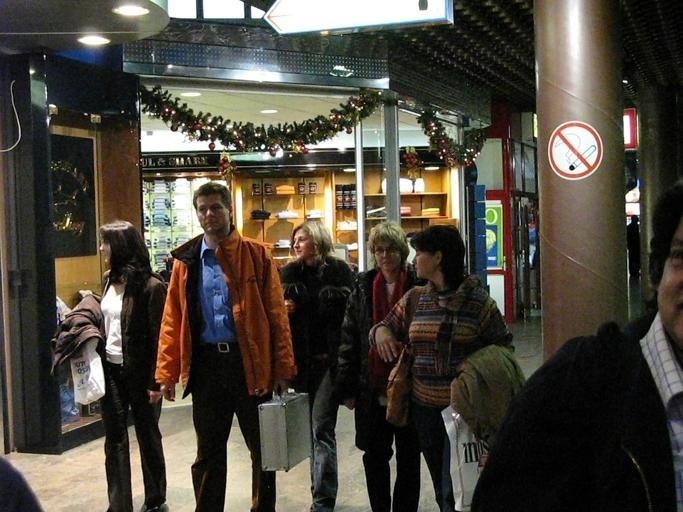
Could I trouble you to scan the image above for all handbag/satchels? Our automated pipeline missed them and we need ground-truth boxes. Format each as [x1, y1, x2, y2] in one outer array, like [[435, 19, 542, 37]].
[[440, 404, 492, 512], [69, 338, 106, 405]]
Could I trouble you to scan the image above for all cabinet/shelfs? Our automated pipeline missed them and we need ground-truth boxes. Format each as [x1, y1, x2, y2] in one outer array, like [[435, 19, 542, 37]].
[[241, 177, 324, 263], [360, 170, 450, 241]]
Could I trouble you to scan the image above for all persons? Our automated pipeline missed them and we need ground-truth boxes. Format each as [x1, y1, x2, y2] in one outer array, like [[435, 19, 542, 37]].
[[155, 181, 297, 511], [369, 224, 515, 512], [334, 220, 428, 512], [276, 219, 355, 512], [469, 178, 683, 512], [626, 214, 641, 280], [74, 220, 168, 512]]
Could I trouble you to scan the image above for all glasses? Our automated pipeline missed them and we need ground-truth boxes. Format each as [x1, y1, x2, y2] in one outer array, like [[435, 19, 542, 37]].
[[370, 245, 402, 254]]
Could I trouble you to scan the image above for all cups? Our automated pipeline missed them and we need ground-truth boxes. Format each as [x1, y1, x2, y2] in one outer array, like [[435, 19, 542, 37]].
[[253, 182, 273, 196], [298, 181, 317, 196]]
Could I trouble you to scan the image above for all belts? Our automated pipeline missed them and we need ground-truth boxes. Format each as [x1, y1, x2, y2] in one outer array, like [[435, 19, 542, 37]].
[[190, 341, 242, 353]]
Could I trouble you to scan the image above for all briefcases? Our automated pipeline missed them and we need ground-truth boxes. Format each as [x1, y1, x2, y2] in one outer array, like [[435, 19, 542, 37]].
[[258, 385, 314, 473]]
